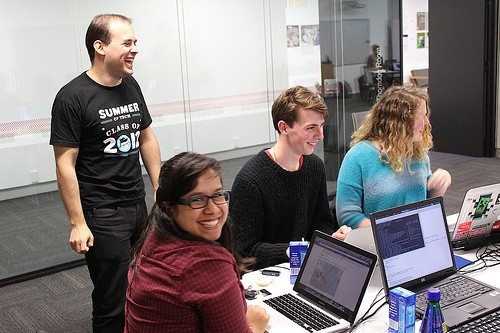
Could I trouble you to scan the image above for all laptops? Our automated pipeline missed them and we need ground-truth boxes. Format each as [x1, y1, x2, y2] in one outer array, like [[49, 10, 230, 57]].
[[382, 59, 401, 71], [246, 182, 500, 333]]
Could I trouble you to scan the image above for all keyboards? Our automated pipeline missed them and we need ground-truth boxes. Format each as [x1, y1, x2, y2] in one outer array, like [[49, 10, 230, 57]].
[[445, 310, 500, 333]]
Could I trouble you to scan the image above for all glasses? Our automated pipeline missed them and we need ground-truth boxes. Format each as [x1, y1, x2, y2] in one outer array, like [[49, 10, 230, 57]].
[[174, 190, 232, 210]]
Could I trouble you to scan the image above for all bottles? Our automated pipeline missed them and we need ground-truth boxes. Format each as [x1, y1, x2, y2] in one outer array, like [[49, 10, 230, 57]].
[[419, 288, 448, 333]]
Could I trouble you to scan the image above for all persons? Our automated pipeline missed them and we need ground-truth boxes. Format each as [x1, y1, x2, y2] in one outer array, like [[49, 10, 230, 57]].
[[367, 44, 383, 67], [335, 85, 452, 229], [50, 13, 162, 333], [123, 151, 271, 333], [228, 85, 353, 269], [311, 25, 320, 46]]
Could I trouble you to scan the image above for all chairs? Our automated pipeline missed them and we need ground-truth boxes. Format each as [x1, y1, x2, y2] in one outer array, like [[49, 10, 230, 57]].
[[360, 59, 398, 107]]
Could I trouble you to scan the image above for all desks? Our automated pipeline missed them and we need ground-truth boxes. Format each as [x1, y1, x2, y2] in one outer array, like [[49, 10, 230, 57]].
[[241, 212, 500, 333]]
